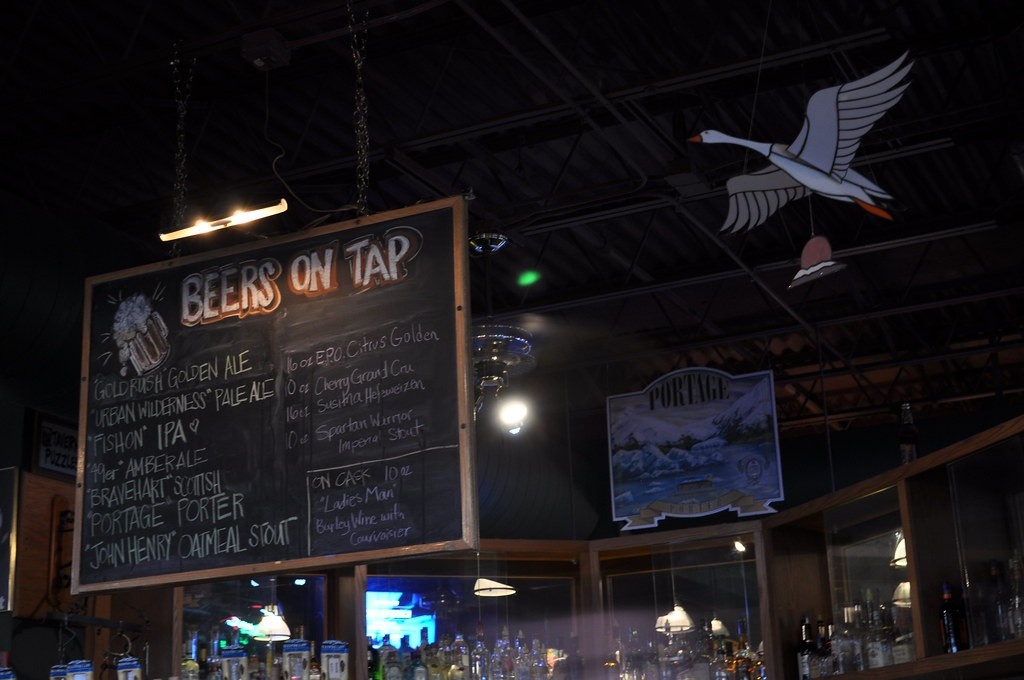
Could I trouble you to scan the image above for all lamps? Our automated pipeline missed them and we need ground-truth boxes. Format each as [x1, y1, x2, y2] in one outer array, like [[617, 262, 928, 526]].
[[474, 228, 535, 420], [253, 591, 293, 643], [892, 581, 912, 608], [891, 539, 908, 567], [655, 548, 696, 638], [473, 578, 516, 598]]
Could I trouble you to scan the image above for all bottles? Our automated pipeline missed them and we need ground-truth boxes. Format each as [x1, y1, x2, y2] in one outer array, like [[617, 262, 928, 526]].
[[309, 641, 321, 680], [367, 621, 548, 680], [179, 625, 223, 680], [796, 589, 916, 680], [610, 620, 768, 680], [941, 548, 1024, 654]]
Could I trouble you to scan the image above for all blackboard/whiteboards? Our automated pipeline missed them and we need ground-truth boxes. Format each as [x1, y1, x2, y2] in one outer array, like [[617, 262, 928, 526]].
[[70, 195, 480, 592]]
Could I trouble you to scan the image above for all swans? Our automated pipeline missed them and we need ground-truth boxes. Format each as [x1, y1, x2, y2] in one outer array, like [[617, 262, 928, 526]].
[[687, 47, 916, 234]]
